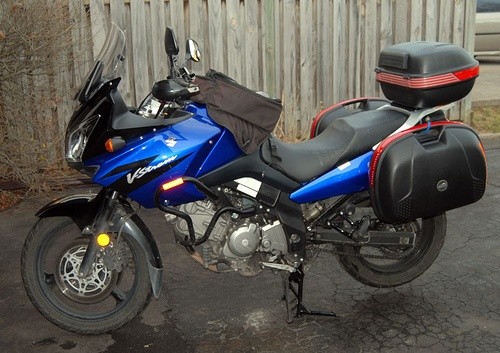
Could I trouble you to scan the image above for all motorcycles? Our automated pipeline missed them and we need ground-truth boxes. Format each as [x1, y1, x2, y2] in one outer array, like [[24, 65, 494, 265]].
[[20, 19, 488, 336]]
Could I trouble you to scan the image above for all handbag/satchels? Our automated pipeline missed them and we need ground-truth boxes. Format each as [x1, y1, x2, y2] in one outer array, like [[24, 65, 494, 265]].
[[189, 67, 283, 153]]
